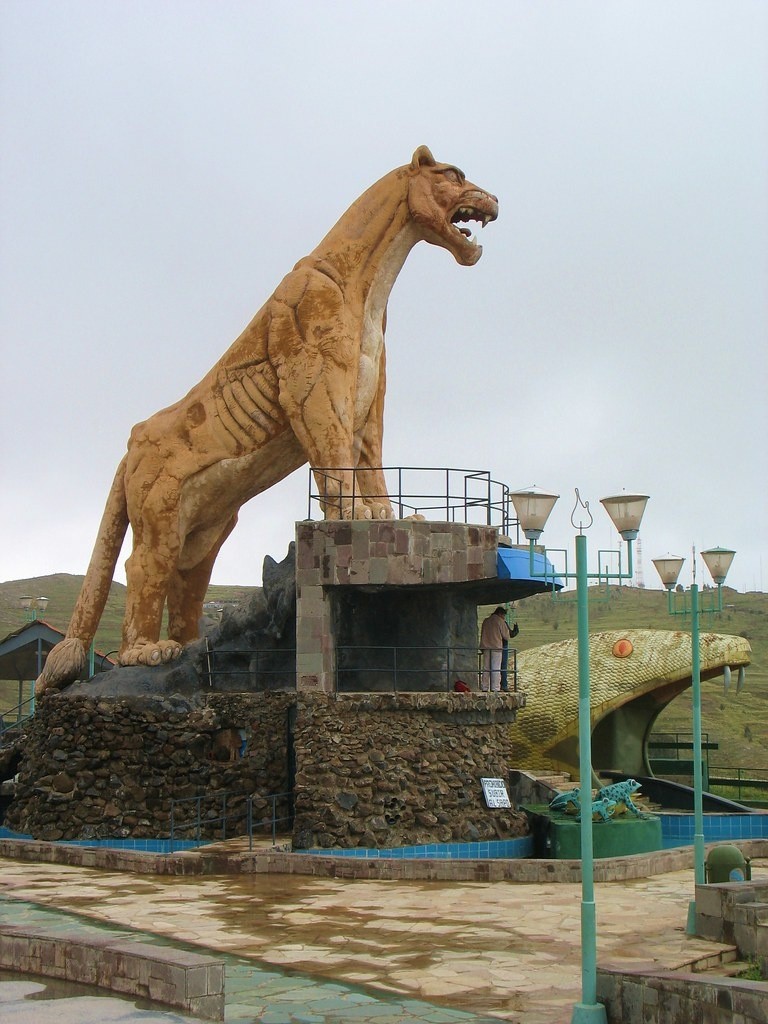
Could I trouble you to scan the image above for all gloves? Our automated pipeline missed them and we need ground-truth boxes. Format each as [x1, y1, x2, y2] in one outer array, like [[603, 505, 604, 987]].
[[514, 622, 518, 630]]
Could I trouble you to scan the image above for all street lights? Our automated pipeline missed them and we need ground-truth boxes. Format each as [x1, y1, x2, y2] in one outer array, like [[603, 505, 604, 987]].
[[507, 483, 653, 1024], [18, 594, 50, 716], [652, 540, 736, 938]]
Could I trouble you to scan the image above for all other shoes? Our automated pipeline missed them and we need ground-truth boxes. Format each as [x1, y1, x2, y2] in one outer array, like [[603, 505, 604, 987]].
[[501, 687, 512, 692]]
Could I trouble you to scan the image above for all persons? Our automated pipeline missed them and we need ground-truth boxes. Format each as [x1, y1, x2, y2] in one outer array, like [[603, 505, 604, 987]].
[[500, 620, 521, 692], [478, 607, 511, 693]]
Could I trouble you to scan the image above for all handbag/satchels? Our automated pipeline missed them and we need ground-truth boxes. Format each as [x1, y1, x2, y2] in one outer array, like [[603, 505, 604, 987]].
[[454, 679, 470, 692]]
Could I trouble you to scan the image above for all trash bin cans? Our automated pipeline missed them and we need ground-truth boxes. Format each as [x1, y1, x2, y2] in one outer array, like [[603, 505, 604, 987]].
[[704, 845, 751, 884]]
